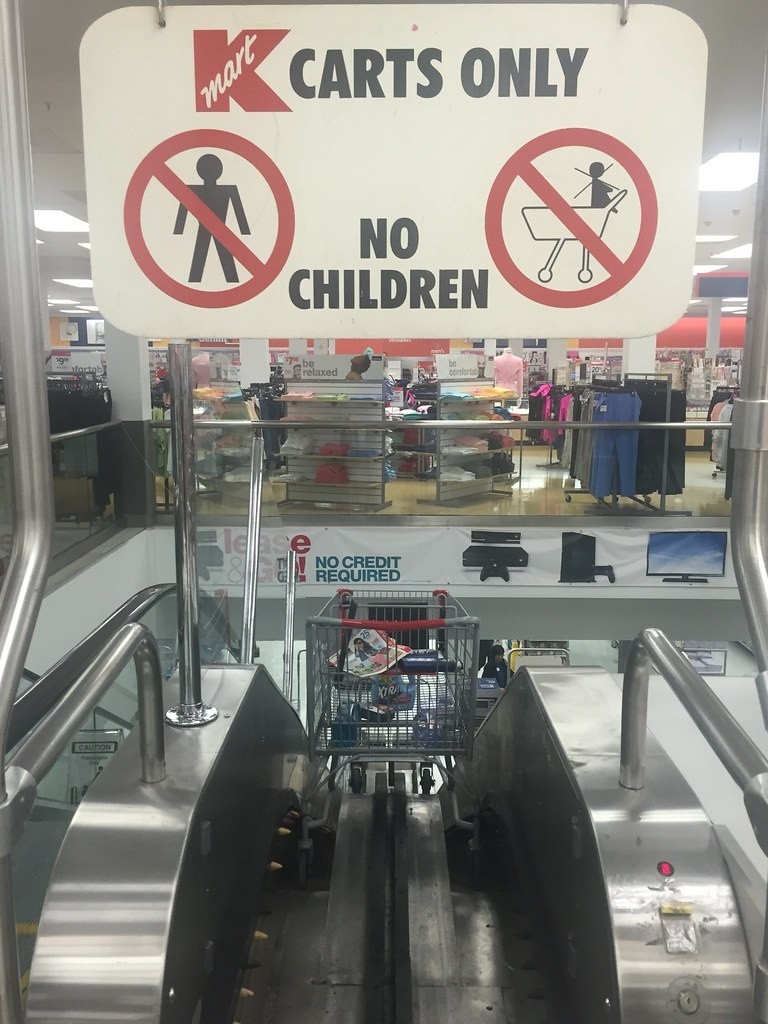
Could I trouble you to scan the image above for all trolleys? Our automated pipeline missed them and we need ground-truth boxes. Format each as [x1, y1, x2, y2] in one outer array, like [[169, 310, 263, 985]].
[[305, 588, 480, 793]]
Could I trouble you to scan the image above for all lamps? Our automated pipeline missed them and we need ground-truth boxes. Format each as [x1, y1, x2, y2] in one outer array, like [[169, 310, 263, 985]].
[[700, 140, 761, 191], [31, 105, 88, 232]]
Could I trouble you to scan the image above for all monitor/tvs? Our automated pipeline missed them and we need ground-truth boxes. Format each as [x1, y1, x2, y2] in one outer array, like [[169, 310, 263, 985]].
[[646, 531, 727, 583]]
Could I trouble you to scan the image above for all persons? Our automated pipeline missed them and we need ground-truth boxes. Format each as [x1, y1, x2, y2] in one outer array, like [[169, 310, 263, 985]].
[[482, 645, 514, 689], [353, 637, 380, 668], [346, 355, 371, 379], [494, 348, 523, 407]]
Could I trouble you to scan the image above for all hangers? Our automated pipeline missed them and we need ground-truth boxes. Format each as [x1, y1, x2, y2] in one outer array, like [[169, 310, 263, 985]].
[[584, 376, 671, 394]]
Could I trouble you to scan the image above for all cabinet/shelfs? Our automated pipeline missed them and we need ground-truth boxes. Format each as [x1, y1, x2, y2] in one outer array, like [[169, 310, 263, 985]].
[[275, 353, 396, 512], [387, 352, 521, 507]]
